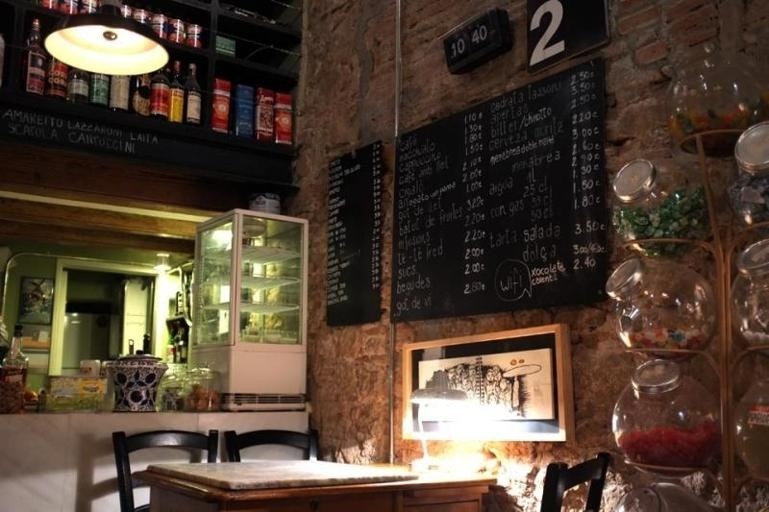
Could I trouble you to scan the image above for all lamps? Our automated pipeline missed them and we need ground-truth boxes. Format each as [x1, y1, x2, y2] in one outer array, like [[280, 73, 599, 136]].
[[411, 370, 474, 475], [38, 5, 169, 78]]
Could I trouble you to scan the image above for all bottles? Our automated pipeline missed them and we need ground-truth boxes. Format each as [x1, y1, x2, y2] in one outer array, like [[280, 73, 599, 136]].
[[725, 121, 768, 227], [143, 334, 151, 354], [611, 159, 724, 264], [156, 366, 223, 413], [606, 257, 720, 365], [663, 40, 764, 159], [726, 352, 768, 482], [722, 236, 768, 350], [1, 325, 30, 387], [166, 335, 187, 364], [20, 18, 203, 129], [610, 355, 724, 482]]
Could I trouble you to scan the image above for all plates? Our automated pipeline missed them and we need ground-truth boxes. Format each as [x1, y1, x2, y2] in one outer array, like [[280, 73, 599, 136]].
[[231, 223, 266, 237]]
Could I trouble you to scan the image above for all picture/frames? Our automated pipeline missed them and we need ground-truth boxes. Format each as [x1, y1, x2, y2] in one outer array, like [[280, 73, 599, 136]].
[[18, 276, 55, 325], [401, 324, 576, 447]]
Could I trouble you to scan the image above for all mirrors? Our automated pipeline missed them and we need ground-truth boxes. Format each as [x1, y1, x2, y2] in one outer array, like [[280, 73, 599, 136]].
[[60, 266, 156, 377]]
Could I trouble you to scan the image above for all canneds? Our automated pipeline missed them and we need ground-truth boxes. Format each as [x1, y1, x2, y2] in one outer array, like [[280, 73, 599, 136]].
[[39, 0, 202, 48]]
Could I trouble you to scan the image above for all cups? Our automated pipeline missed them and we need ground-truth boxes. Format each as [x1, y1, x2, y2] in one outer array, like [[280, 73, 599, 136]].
[[79, 360, 101, 377]]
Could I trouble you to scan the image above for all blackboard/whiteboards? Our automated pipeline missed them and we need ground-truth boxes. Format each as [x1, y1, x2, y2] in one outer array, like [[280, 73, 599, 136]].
[[390, 57, 610, 323], [324, 138, 384, 327]]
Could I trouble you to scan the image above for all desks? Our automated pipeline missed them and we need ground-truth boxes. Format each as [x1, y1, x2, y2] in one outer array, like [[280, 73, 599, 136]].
[[132, 459, 496, 512]]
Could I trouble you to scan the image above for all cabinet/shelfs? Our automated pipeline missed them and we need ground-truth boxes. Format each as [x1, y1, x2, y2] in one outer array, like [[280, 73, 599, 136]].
[[22, 1, 301, 153], [190, 217, 305, 349]]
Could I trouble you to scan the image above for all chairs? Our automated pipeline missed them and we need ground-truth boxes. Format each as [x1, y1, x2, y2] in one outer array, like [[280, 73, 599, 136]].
[[111, 430, 218, 510], [226, 428, 317, 461]]
